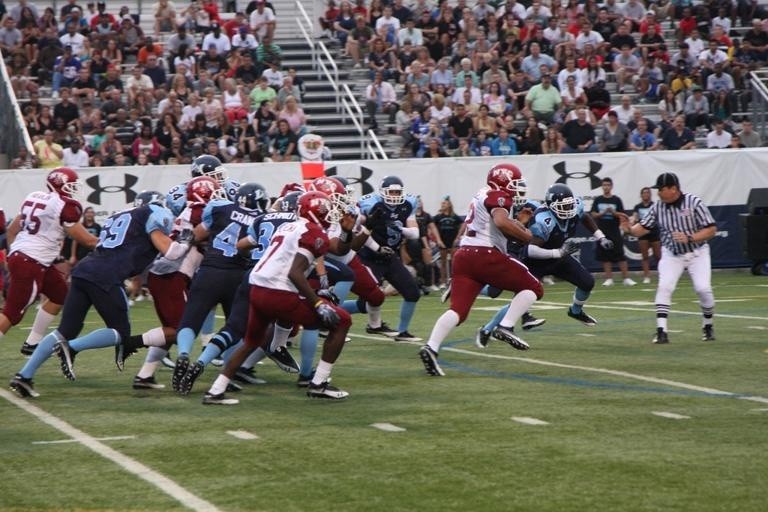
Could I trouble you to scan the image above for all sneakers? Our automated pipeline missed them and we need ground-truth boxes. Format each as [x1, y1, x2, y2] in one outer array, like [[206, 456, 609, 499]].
[[308, 382, 349, 400], [700, 323, 715, 342], [567, 306, 598, 327], [132, 376, 166, 389], [395, 331, 423, 343], [12, 372, 40, 399], [365, 322, 400, 337], [423, 284, 451, 302], [20, 341, 39, 359], [297, 371, 332, 387], [653, 329, 669, 344], [522, 317, 546, 330], [476, 327, 490, 348], [53, 341, 78, 382], [604, 277, 652, 286], [173, 343, 267, 407], [419, 345, 446, 377], [267, 343, 300, 373], [492, 328, 531, 350], [115, 339, 137, 372]]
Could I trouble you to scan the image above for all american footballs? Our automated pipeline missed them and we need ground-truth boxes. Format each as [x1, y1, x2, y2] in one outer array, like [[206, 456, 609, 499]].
[[500, 218, 527, 244]]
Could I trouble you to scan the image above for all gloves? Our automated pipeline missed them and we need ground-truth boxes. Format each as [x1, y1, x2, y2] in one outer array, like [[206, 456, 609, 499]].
[[385, 222, 405, 234], [176, 229, 196, 248], [556, 240, 580, 258], [594, 232, 614, 254]]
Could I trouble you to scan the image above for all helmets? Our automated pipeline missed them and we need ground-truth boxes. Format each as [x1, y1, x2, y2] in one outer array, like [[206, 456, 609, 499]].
[[280, 191, 304, 212], [134, 190, 162, 209], [188, 177, 220, 205], [46, 168, 79, 198], [297, 191, 331, 228], [191, 154, 228, 184], [546, 183, 579, 221], [236, 182, 268, 214], [308, 176, 347, 220], [486, 164, 530, 208], [382, 176, 406, 205]]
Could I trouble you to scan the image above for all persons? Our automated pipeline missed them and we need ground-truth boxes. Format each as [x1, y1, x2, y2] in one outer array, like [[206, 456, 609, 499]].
[[1, 155, 717, 405], [2, 1, 308, 168], [319, 1, 767, 158]]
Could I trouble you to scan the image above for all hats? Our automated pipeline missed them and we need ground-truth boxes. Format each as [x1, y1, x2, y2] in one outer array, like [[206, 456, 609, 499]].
[[650, 172, 680, 187]]
